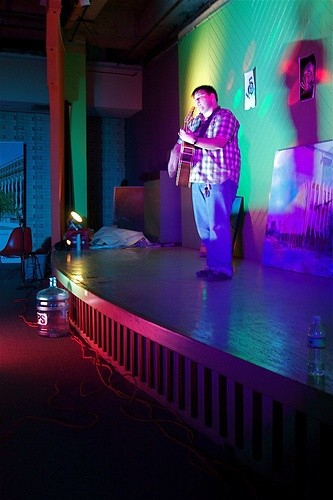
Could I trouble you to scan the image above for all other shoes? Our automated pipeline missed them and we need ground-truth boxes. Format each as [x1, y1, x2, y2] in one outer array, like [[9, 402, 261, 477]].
[[196, 269, 232, 282]]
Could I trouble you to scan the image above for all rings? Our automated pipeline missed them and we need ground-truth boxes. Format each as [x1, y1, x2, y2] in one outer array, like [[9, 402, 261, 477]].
[[178, 132, 182, 135]]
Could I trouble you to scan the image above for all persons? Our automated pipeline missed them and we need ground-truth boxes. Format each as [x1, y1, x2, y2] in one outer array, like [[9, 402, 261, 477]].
[[176, 84, 242, 283]]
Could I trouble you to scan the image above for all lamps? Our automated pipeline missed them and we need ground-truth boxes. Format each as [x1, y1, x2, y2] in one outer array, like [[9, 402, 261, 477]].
[[63, 211, 87, 251]]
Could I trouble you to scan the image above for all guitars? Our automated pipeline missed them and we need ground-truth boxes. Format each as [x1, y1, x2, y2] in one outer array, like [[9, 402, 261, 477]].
[[174, 108, 196, 189]]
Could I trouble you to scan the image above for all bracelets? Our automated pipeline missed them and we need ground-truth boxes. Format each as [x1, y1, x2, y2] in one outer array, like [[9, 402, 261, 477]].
[[193, 137, 199, 145]]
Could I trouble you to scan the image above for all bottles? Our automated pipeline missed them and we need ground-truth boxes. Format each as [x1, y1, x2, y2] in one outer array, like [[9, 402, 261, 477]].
[[36, 277, 70, 338], [305, 315, 326, 385]]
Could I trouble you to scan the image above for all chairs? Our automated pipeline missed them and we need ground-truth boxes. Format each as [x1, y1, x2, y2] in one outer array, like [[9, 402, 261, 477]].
[[0, 226, 42, 289]]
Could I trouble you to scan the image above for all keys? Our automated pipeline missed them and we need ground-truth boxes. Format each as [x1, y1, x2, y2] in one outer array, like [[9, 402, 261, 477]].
[[203, 184, 212, 197]]
